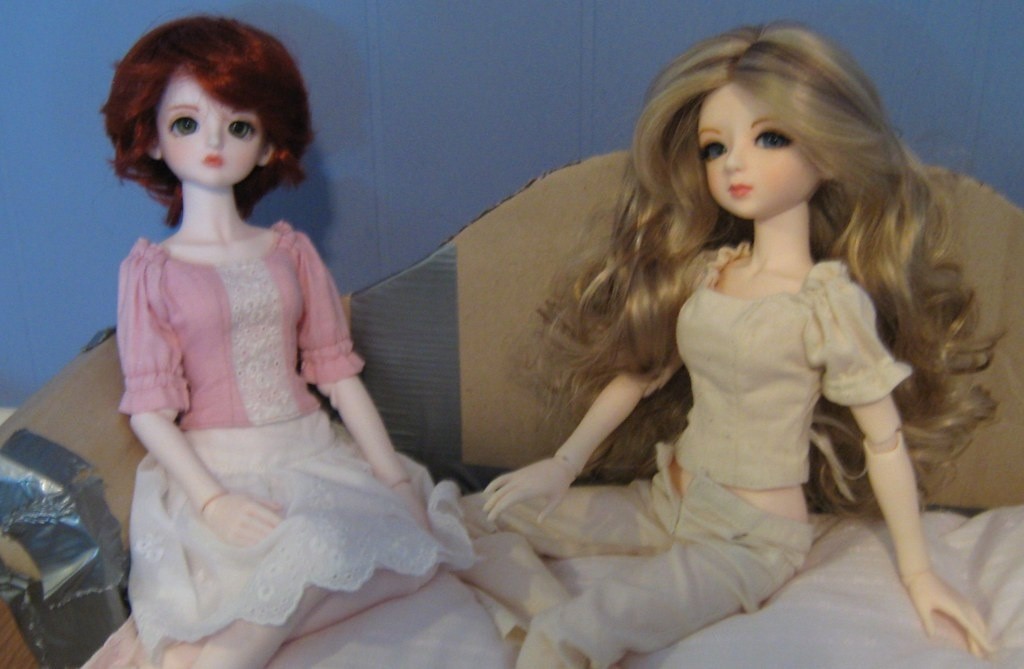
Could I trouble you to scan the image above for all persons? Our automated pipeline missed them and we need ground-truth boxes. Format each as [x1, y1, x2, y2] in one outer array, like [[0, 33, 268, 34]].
[[442, 22, 994, 669], [101, 18, 487, 669]]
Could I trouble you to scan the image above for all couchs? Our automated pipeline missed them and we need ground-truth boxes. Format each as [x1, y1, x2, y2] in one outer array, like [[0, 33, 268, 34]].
[[2, 139, 1024, 669]]
